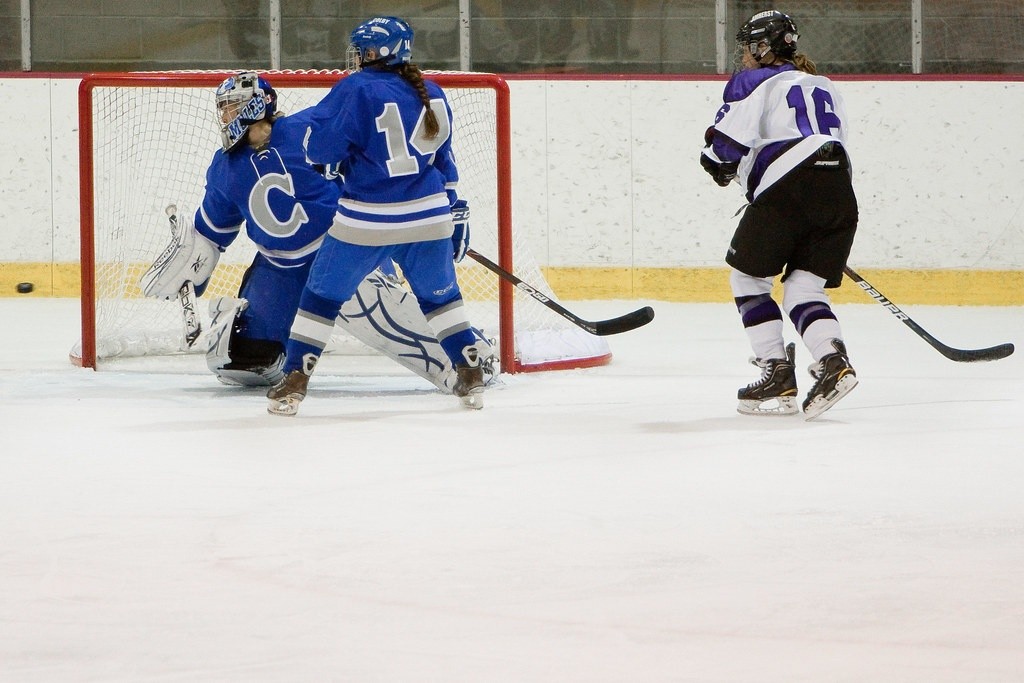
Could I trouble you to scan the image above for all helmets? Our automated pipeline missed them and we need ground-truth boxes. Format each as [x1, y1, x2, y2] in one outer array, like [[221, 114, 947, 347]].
[[734, 10, 800, 69], [213, 71, 278, 155], [349, 15, 415, 72]]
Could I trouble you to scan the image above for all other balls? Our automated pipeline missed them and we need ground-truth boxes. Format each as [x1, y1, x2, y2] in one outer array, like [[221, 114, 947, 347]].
[[17, 283, 34, 293]]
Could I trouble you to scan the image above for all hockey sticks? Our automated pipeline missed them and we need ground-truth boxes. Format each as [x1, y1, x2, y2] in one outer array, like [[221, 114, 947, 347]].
[[842, 265, 1014, 365], [465, 246, 655, 337], [166, 204, 239, 349]]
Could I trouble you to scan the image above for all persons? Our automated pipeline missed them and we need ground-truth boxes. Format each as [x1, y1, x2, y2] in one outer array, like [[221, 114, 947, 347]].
[[139, 72, 493, 400], [699, 9, 863, 424], [263, 11, 487, 409]]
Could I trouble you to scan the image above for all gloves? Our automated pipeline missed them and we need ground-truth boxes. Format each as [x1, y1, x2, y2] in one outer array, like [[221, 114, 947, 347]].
[[451, 199, 472, 265], [700, 151, 742, 188]]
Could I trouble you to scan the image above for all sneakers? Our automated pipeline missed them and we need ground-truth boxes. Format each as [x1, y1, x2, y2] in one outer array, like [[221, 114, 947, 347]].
[[802, 338, 860, 424], [265, 368, 310, 416], [450, 356, 486, 411], [736, 342, 799, 416]]
